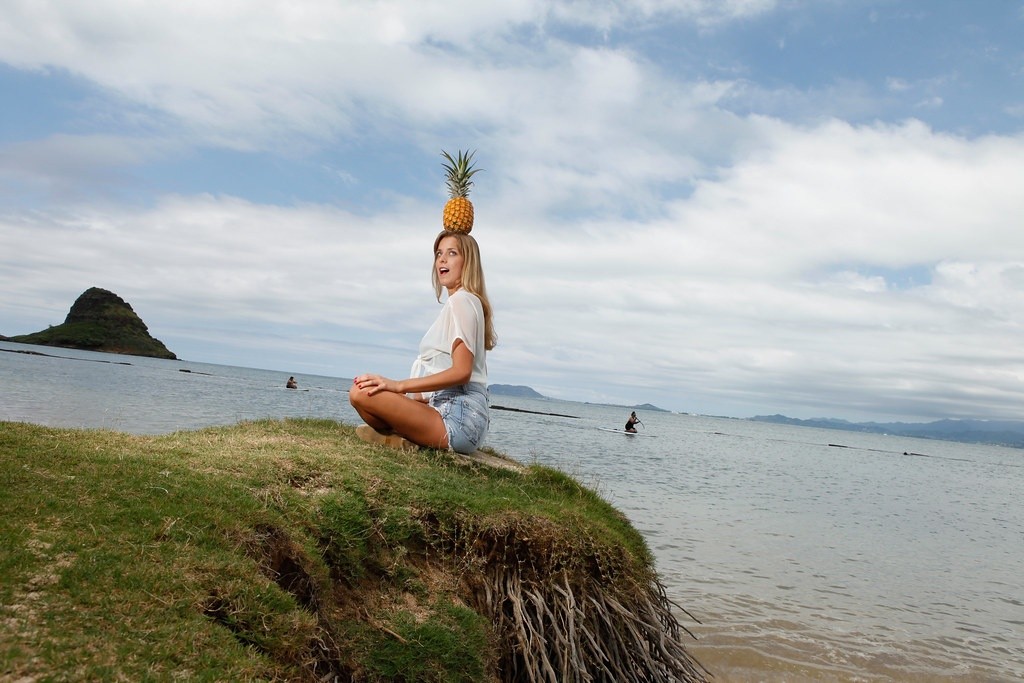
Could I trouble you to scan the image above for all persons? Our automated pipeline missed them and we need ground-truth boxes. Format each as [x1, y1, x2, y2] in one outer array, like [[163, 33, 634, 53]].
[[348, 231, 499, 455], [624, 411, 640, 434], [286, 376, 297, 391]]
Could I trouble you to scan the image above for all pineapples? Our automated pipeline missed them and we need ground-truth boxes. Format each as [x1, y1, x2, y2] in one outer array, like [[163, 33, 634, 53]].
[[439, 148, 484, 234]]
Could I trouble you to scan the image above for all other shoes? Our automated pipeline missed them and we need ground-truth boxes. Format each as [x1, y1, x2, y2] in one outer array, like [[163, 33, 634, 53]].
[[356, 425, 419, 452]]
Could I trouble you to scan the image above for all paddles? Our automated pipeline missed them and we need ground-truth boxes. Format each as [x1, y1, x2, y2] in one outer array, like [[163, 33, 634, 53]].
[[633, 415, 645, 429]]
